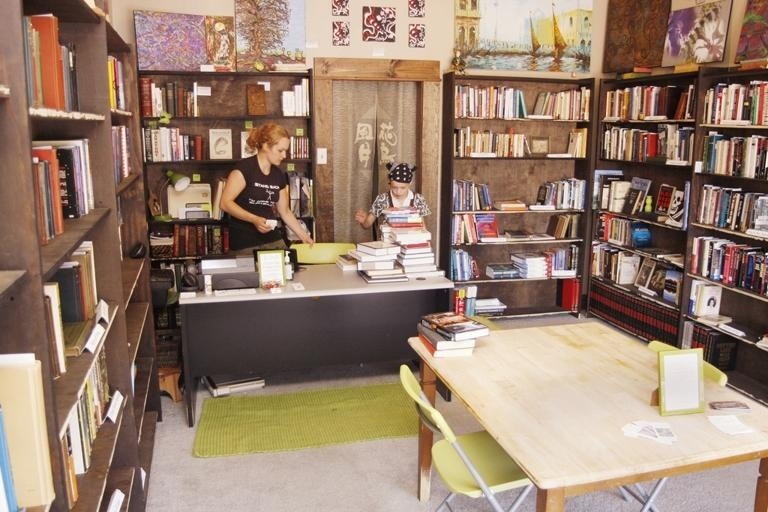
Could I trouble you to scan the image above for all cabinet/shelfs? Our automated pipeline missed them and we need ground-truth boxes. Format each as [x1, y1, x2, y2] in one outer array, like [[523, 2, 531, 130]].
[[0, 2, 165, 512], [143, 70, 312, 366], [443, 73, 592, 323], [593, 73, 700, 347], [687, 65, 767, 410]]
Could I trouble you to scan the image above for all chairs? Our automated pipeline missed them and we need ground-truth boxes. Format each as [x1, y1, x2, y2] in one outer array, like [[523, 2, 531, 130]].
[[287, 242, 358, 267], [398, 364, 538, 511], [614, 340, 728, 511]]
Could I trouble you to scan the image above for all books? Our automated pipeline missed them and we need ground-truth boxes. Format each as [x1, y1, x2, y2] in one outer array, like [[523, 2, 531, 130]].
[[148, 223, 229, 258], [286, 173, 310, 217], [422, 312, 488, 340], [204, 375, 265, 397], [159, 264, 204, 343], [338, 207, 445, 283], [241, 131, 258, 160], [210, 176, 228, 221], [0, 13, 151, 511], [281, 78, 309, 116], [246, 84, 266, 114], [451, 82, 590, 315], [290, 136, 310, 160], [418, 332, 474, 357], [592, 83, 768, 351], [139, 76, 202, 163], [417, 323, 476, 350], [208, 128, 232, 161]]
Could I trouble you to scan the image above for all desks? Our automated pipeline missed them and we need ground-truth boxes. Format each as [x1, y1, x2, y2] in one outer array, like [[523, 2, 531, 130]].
[[403, 319, 766, 511], [175, 258, 457, 425]]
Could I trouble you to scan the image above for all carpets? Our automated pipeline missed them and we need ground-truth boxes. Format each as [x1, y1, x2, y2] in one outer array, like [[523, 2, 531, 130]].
[[189, 383, 421, 458]]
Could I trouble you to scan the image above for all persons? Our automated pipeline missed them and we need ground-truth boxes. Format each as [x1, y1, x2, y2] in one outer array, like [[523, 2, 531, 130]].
[[220, 124, 312, 259], [355, 161, 432, 229]]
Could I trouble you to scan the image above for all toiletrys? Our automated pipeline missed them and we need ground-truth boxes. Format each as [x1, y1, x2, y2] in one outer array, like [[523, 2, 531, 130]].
[[284, 250, 294, 281]]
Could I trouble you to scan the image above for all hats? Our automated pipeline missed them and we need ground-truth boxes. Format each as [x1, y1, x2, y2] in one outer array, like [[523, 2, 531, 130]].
[[386, 162, 417, 183]]
